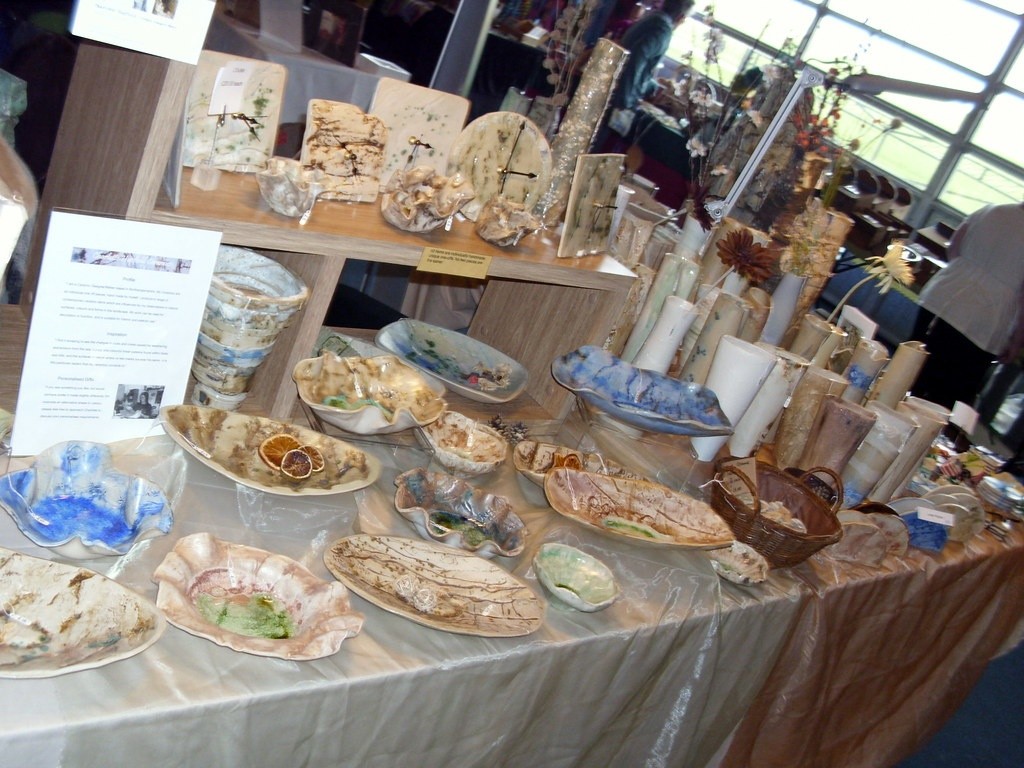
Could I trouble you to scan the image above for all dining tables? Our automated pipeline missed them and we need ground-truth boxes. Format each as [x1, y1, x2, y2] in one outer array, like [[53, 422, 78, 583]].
[[0, 0, 1024, 768]]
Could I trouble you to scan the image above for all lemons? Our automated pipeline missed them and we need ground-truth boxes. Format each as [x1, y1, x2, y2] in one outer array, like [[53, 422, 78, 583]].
[[259, 433, 324, 480]]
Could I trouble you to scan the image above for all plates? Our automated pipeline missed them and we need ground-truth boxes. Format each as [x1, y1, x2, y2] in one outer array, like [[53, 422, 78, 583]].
[[414, 410, 507, 479], [543, 466, 735, 550], [323, 534, 546, 638], [551, 343, 734, 438], [192, 247, 308, 410], [394, 467, 529, 559], [513, 440, 656, 489], [319, 331, 447, 398], [0, 548, 166, 679], [1, 440, 174, 559], [373, 317, 528, 403], [159, 405, 381, 495], [706, 538, 769, 586], [291, 347, 447, 435], [531, 543, 620, 612], [150, 531, 366, 662]]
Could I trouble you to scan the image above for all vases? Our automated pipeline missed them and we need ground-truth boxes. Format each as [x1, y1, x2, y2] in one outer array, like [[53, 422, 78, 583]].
[[635, 208, 948, 522]]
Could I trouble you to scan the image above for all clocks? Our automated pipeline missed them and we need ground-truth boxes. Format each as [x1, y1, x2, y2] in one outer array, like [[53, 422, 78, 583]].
[[357, 78, 473, 206], [184, 48, 286, 179], [443, 109, 553, 236], [553, 150, 621, 258], [302, 93, 384, 203]]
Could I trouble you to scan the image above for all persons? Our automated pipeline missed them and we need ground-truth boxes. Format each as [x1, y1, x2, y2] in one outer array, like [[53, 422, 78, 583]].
[[586, 0, 695, 154], [905, 201, 1024, 413], [123, 391, 152, 420]]
